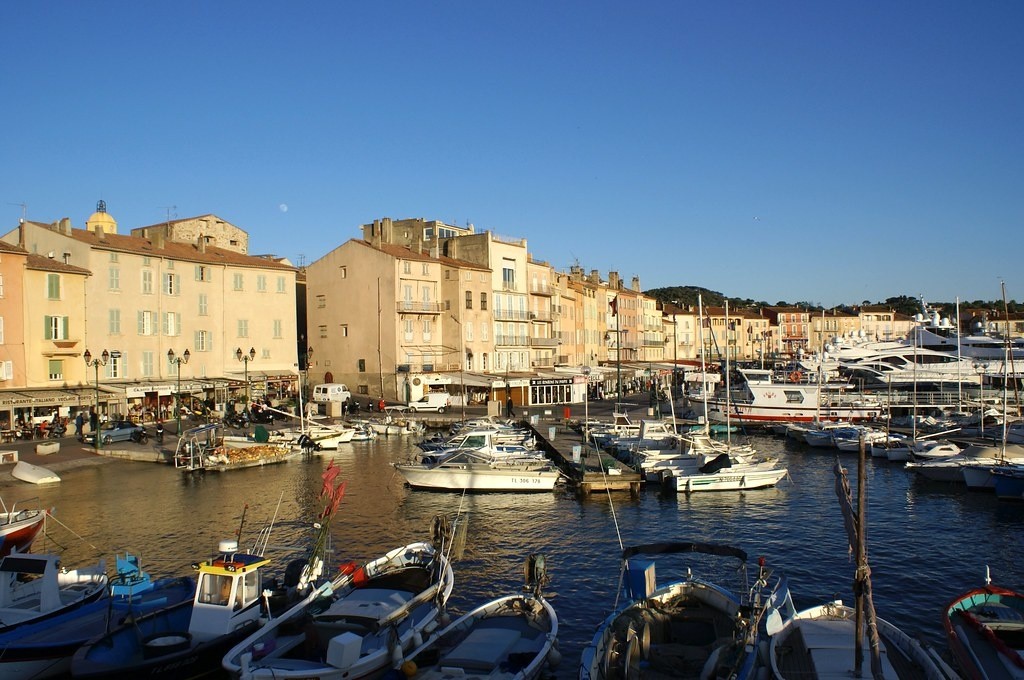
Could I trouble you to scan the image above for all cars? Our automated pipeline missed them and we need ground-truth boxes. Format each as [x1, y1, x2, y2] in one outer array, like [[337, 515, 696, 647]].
[[82, 420, 146, 445]]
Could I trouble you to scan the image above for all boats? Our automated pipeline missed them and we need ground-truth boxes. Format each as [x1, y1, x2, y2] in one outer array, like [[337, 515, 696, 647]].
[[0, 416, 1024, 680]]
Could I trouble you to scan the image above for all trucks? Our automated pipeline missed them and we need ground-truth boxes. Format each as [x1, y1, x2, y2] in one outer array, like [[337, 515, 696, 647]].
[[409, 392, 452, 413]]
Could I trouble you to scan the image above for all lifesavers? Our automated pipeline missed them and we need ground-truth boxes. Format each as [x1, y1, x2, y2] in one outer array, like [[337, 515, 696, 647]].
[[789, 371, 801, 383]]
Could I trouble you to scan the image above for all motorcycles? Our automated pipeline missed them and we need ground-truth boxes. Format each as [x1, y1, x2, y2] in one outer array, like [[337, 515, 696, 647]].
[[221, 401, 289, 431], [130, 429, 148, 446], [156, 424, 164, 443], [341, 398, 375, 416]]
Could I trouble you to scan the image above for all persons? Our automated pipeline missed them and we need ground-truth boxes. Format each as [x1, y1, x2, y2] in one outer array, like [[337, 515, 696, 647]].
[[15, 411, 97, 439], [128, 381, 314, 431], [507, 397, 516, 418], [590, 375, 690, 400]]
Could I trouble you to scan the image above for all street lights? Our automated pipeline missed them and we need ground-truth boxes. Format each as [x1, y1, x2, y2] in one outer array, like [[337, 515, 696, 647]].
[[604, 329, 621, 414], [745, 342, 754, 360], [703, 342, 713, 373], [166, 348, 191, 436], [304, 346, 314, 404], [728, 339, 737, 371], [665, 334, 678, 401], [84, 348, 109, 449], [236, 347, 256, 416]]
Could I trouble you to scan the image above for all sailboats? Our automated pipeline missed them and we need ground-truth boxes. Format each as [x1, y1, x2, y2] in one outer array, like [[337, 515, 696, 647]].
[[631, 293, 789, 492], [770, 279, 1024, 502]]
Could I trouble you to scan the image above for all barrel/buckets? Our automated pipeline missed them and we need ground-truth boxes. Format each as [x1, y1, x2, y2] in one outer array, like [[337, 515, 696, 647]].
[[142, 632, 192, 661]]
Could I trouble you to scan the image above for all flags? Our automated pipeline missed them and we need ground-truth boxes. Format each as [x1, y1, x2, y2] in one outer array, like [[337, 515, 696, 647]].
[[609, 296, 618, 317], [761, 331, 772, 337], [727, 322, 737, 331], [702, 317, 711, 329], [747, 326, 754, 334]]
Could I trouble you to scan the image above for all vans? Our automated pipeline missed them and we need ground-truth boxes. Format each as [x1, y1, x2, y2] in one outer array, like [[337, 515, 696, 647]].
[[313, 383, 352, 404]]
